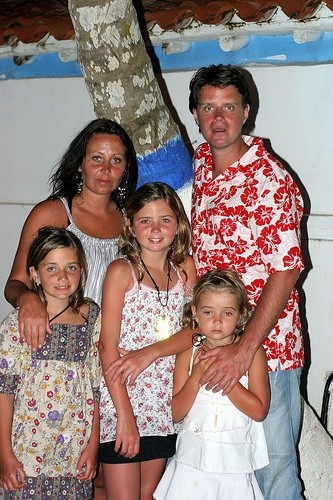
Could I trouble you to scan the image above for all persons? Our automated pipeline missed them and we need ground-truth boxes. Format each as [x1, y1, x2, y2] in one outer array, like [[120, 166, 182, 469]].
[[96, 182, 194, 500], [3, 118, 138, 348], [0, 225, 103, 500], [186, 63, 308, 500], [148, 270, 271, 500]]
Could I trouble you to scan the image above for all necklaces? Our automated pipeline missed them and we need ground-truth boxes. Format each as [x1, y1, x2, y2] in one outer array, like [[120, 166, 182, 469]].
[[191, 329, 239, 354], [50, 304, 71, 322], [139, 252, 171, 306]]
